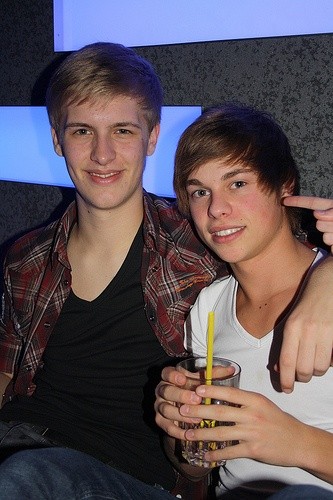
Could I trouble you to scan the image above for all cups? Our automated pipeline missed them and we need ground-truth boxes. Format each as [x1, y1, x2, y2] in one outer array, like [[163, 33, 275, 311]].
[[176, 355, 241, 470]]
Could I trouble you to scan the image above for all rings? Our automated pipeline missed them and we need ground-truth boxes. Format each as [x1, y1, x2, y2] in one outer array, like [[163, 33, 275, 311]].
[[157, 382, 172, 399]]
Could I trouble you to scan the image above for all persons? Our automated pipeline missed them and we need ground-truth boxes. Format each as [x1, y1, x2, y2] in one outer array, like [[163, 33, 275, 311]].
[[0, 39, 332, 499], [281, 195, 332, 254], [0, 106, 333, 500]]
